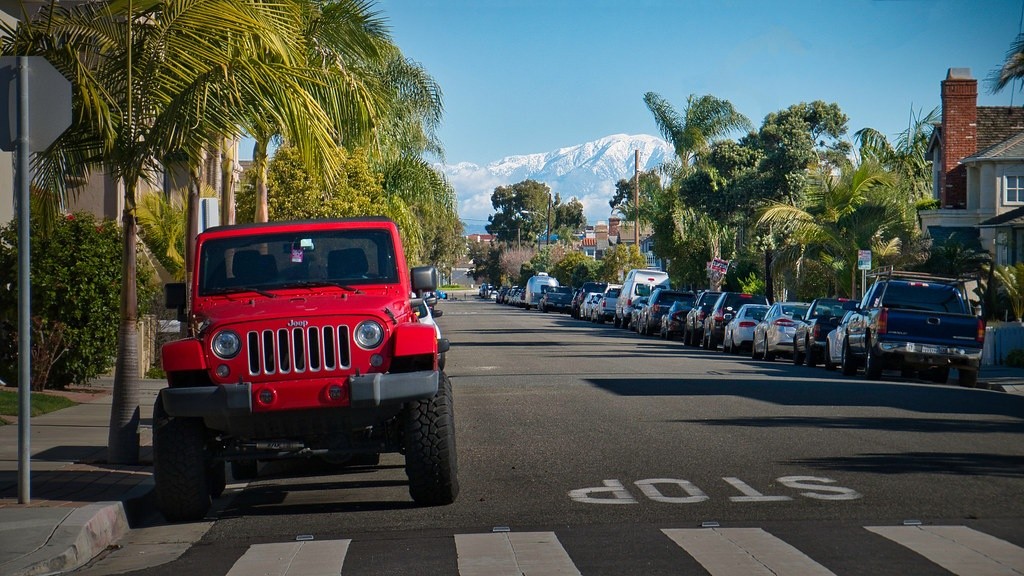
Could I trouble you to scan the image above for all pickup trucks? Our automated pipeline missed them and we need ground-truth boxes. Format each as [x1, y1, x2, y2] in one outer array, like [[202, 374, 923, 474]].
[[793, 298, 860, 367], [841, 264, 985, 387]]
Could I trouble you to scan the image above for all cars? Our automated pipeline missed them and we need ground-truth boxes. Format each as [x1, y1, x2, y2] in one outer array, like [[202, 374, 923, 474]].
[[824, 303, 860, 370], [637, 289, 695, 335], [751, 302, 829, 362], [683, 292, 724, 347], [724, 304, 772, 354], [628, 297, 648, 331], [702, 294, 768, 349], [660, 300, 696, 340]]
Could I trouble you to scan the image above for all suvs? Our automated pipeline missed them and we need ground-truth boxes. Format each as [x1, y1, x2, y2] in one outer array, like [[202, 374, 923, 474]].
[[152, 218, 460, 517]]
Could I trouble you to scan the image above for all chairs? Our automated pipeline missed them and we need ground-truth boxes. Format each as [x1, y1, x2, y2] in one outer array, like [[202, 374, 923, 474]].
[[230, 244, 370, 290]]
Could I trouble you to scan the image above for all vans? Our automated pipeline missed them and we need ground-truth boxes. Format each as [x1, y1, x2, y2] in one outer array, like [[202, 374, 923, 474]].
[[476, 270, 623, 324], [612, 268, 669, 328]]
